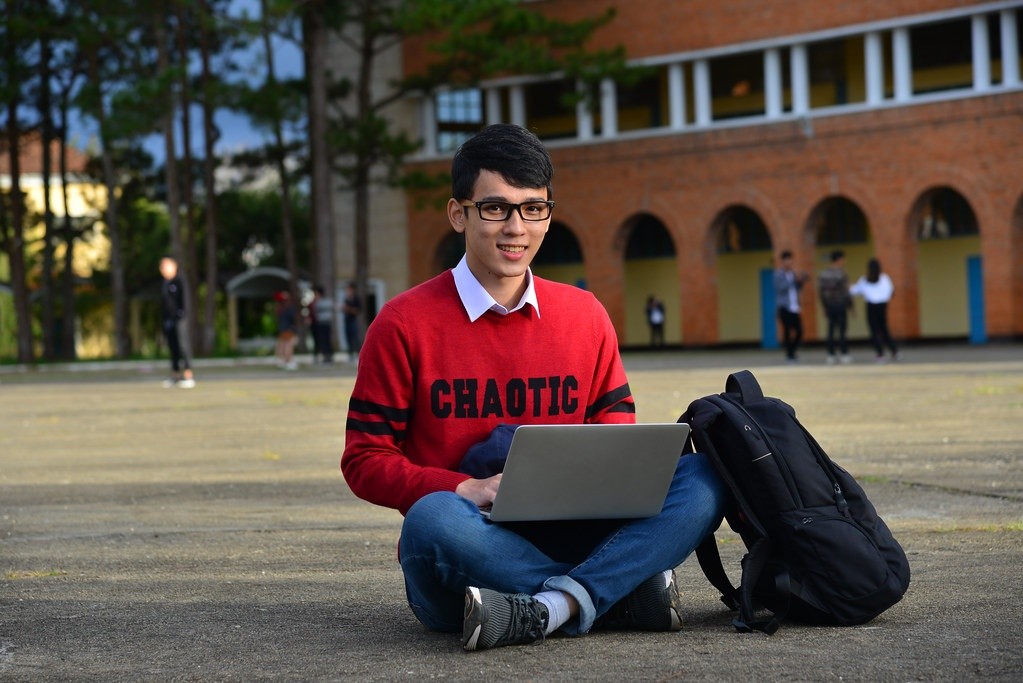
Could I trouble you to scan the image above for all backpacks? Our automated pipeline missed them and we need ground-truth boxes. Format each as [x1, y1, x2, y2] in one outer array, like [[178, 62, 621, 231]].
[[676, 370, 911, 630]]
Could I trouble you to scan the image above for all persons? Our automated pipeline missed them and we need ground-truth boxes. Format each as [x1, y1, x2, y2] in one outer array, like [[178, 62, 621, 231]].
[[160, 258, 195, 388], [308, 286, 340, 363], [274, 291, 296, 369], [820, 250, 853, 364], [772, 251, 806, 360], [646, 298, 665, 346], [340, 123, 717, 651], [848, 261, 901, 361], [340, 283, 362, 361]]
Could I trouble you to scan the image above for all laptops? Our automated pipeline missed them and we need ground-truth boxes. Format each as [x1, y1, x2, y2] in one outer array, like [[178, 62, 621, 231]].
[[479, 423, 690, 522]]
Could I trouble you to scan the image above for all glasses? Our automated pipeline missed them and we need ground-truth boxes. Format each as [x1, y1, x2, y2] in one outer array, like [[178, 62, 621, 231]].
[[461, 197, 553, 222]]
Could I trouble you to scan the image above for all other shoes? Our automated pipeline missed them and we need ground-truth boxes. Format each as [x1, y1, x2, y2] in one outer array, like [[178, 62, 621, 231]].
[[875, 350, 905, 364], [180, 377, 196, 390], [161, 375, 182, 388], [826, 353, 851, 366]]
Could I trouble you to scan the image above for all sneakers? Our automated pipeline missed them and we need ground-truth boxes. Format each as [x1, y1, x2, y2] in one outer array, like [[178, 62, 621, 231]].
[[602, 569, 686, 634], [465, 584, 550, 653]]
